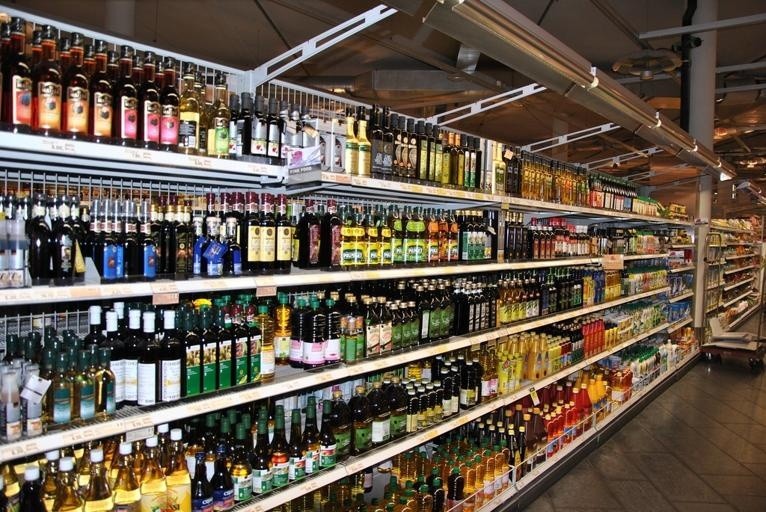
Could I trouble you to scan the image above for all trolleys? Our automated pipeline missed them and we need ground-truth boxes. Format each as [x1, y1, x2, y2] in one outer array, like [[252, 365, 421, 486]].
[[697, 232, 766, 370]]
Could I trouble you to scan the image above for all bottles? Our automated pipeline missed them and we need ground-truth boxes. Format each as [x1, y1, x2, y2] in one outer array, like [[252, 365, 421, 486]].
[[505, 325, 706, 388], [1, 399, 275, 512], [277, 372, 504, 483], [230, 91, 504, 197], [506, 388, 695, 479], [0, 293, 276, 443], [505, 266, 669, 323], [505, 144, 657, 214], [505, 211, 662, 260], [280, 484, 514, 512], [275, 278, 505, 371], [1, 191, 231, 286], [231, 188, 503, 277], [0, 14, 228, 158]]
